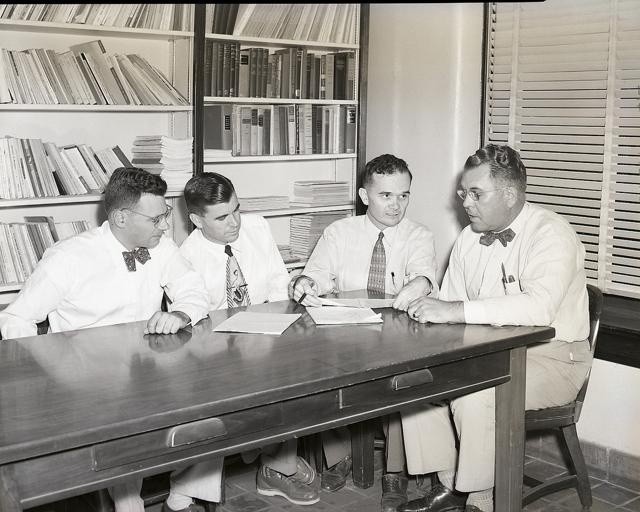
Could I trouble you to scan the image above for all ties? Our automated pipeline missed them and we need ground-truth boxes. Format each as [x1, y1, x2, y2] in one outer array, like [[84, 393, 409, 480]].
[[367, 232, 386, 292], [225, 245, 252, 309]]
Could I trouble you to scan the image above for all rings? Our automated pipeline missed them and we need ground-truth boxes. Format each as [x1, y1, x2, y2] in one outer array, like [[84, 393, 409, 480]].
[[413, 313, 416, 318]]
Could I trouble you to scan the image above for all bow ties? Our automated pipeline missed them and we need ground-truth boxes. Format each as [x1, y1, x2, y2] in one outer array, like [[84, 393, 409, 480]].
[[122, 248, 152, 272], [479, 227, 516, 247]]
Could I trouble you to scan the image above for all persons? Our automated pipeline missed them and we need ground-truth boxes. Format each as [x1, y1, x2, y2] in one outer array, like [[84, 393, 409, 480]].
[[292, 155, 440, 507], [399, 143, 591, 508], [163, 172, 322, 512], [1, 166, 225, 511]]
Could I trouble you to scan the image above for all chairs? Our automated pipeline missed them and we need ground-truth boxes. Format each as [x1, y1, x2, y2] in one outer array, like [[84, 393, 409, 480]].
[[523, 283, 595, 507]]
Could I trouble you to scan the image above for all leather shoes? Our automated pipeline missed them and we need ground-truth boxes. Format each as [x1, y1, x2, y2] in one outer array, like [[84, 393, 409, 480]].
[[256, 465, 321, 505], [163, 498, 205, 512], [381, 468, 409, 512], [287, 456, 315, 485], [321, 458, 353, 492], [396, 482, 468, 512]]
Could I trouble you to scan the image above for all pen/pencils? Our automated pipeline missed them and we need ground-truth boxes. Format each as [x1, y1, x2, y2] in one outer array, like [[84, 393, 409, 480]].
[[292, 282, 315, 312], [509, 274, 517, 286], [502, 262, 508, 283], [502, 277, 508, 290]]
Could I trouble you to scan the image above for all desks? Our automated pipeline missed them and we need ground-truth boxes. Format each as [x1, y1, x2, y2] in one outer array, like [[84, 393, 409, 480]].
[[0, 288, 557, 509]]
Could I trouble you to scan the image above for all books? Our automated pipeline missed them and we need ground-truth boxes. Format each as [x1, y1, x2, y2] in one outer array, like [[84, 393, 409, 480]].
[[2, 2, 186, 32], [237, 178, 351, 208], [286, 214, 353, 261], [204, 107, 358, 156], [4, 133, 193, 199], [212, 4, 358, 45], [204, 42, 354, 101], [2, 39, 188, 107], [3, 212, 94, 286]]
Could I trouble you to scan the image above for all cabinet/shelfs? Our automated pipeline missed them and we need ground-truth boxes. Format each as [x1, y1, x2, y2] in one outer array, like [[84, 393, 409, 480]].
[[0, 0, 201, 293], [202, 1, 371, 271]]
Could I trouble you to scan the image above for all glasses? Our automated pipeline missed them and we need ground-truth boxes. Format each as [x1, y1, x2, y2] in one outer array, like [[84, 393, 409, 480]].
[[456, 189, 508, 201], [121, 203, 173, 228]]
